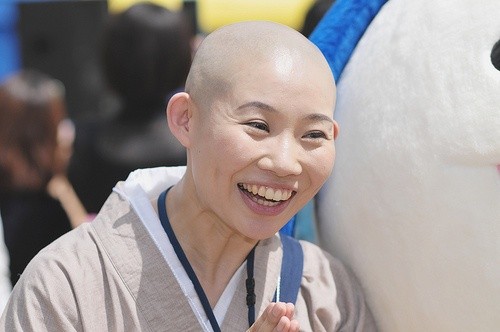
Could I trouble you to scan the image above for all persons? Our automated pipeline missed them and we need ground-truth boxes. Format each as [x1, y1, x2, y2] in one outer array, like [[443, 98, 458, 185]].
[[0, 22, 379, 332], [1, 1, 204, 291]]
